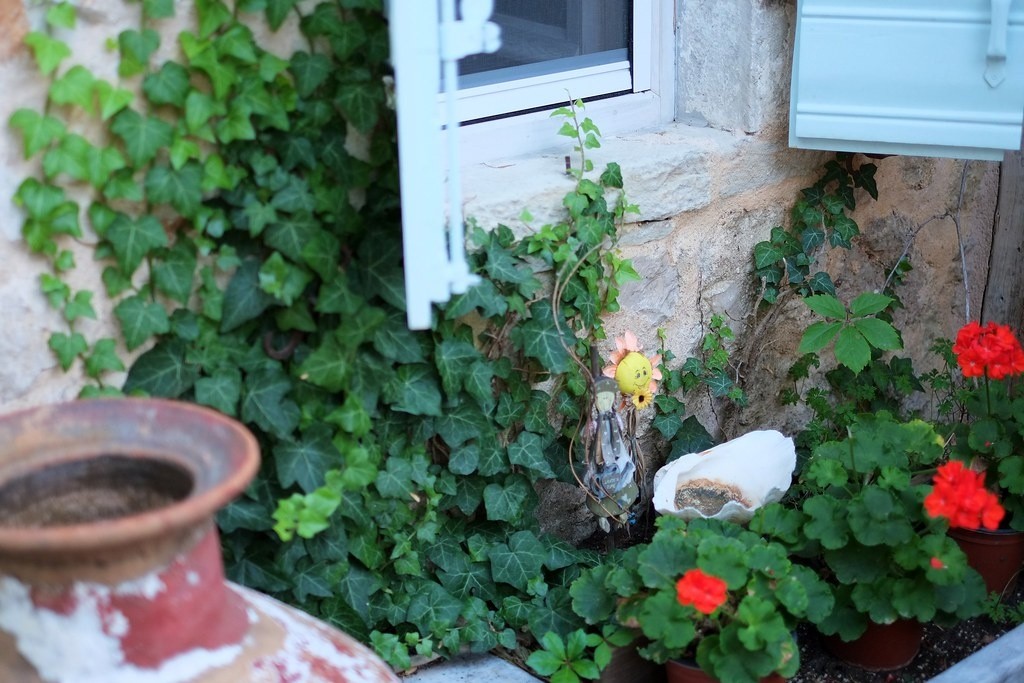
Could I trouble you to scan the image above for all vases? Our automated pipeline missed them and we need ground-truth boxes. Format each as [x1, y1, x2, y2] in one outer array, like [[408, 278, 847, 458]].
[[946, 526, 1024, 601], [665, 626, 786, 683]]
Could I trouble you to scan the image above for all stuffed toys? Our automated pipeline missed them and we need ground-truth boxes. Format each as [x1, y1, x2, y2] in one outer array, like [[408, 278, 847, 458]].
[[579, 376, 639, 532]]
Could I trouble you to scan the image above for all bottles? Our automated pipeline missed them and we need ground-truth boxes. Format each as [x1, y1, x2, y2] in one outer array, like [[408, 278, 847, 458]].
[[0, 398, 400, 683]]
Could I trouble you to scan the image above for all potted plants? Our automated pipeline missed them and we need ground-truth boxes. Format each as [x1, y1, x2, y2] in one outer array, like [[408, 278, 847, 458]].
[[806, 409, 990, 673]]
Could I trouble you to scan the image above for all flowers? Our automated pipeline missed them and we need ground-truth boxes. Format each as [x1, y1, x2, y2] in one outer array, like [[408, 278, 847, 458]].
[[923, 318, 1024, 532], [568, 500, 835, 683]]
